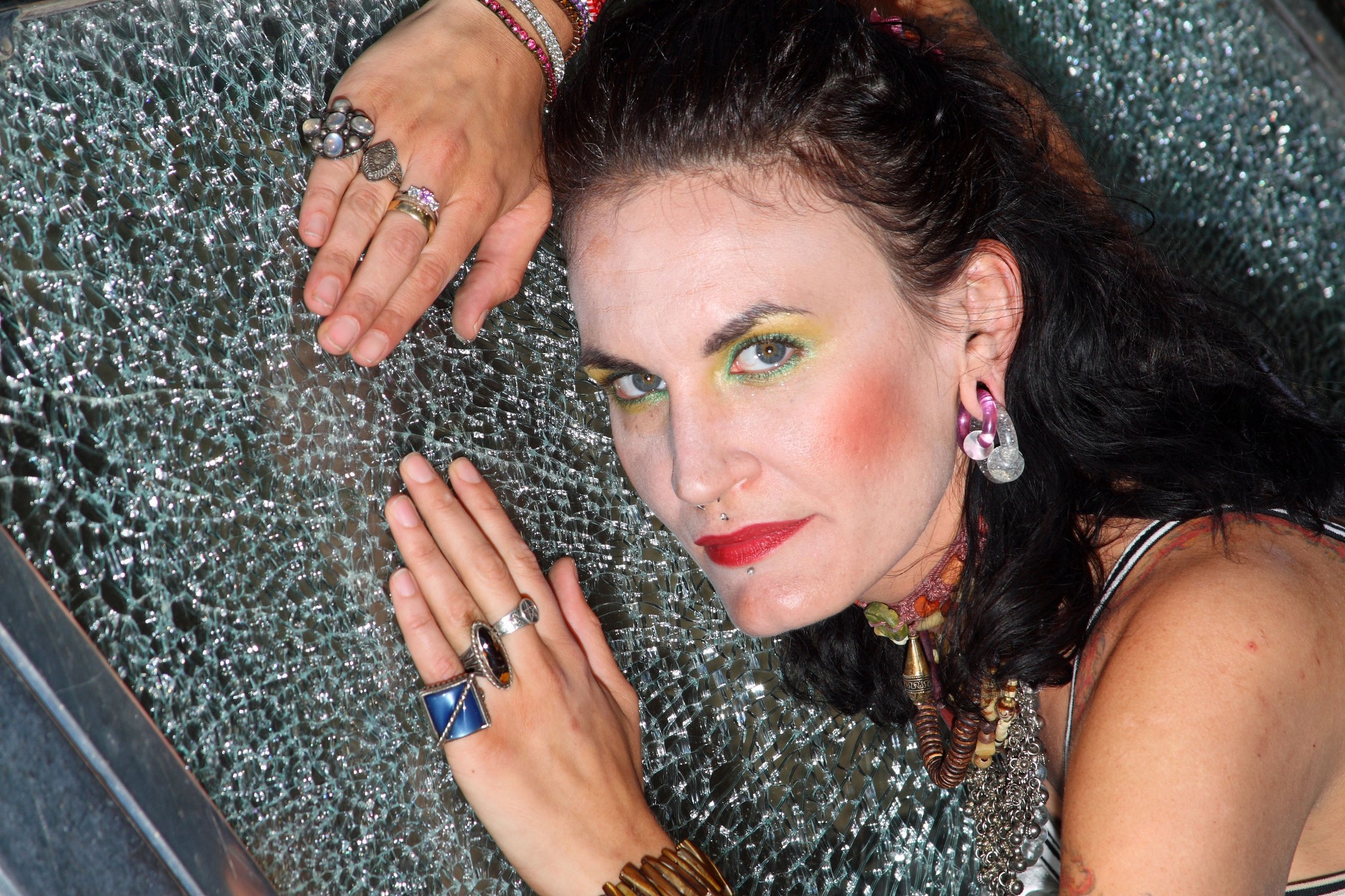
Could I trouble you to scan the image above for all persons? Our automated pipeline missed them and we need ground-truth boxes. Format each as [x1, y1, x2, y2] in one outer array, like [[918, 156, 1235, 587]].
[[298, 0, 1345, 896]]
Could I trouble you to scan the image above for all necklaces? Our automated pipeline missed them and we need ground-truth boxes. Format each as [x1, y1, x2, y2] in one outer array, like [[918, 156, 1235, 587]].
[[852, 511, 1023, 787]]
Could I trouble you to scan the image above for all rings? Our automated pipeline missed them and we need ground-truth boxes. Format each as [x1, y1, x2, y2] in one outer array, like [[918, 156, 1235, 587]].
[[491, 598, 539, 637], [460, 621, 512, 690], [416, 673, 491, 748], [386, 186, 441, 244], [360, 139, 403, 187], [302, 99, 375, 161]]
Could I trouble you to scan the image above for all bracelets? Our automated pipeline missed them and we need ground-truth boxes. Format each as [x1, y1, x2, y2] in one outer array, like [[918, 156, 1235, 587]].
[[599, 839, 735, 896], [476, 0, 605, 114]]
